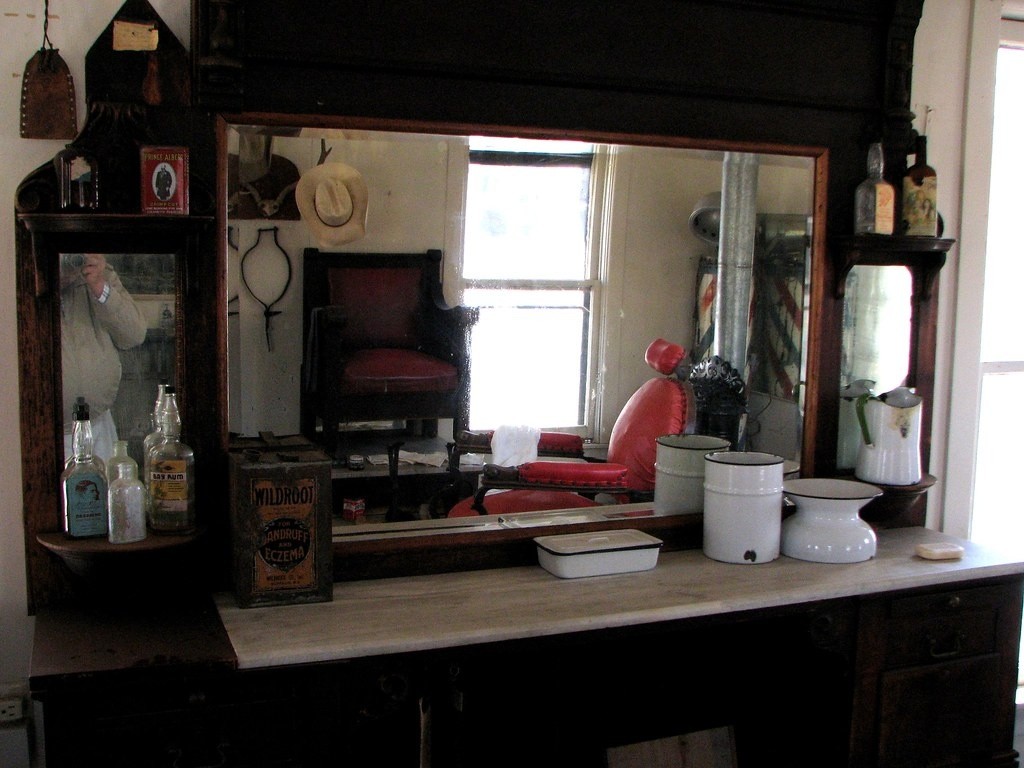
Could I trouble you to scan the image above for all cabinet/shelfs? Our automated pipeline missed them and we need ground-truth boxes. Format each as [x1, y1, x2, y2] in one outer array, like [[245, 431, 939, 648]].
[[212, 571, 1024, 768]]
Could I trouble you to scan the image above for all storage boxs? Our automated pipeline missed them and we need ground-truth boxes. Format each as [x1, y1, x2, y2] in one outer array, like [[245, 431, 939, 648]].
[[138, 143, 193, 216], [231, 428, 314, 454], [230, 449, 337, 614], [531, 526, 663, 579]]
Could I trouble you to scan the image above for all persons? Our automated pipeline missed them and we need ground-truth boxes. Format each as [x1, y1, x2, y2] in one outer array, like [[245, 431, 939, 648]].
[[61, 254, 147, 465]]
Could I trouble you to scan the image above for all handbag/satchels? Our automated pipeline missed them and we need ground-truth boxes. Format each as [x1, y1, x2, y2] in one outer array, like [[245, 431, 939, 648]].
[[20, 49, 78, 141]]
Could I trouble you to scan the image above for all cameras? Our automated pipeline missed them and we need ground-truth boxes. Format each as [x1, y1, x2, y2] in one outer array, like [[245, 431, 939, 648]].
[[59, 254, 85, 270]]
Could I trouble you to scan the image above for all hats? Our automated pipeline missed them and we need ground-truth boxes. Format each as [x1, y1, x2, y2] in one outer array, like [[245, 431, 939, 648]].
[[237, 131, 274, 185], [295, 163, 369, 249]]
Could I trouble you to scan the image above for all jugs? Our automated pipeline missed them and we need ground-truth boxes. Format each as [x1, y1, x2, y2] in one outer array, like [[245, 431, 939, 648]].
[[854, 386, 924, 486]]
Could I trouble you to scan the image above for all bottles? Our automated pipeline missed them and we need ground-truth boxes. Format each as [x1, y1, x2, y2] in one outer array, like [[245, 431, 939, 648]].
[[61, 402, 110, 538], [106, 441, 138, 485], [64, 397, 83, 469], [108, 463, 146, 544], [144, 384, 170, 480], [901, 136, 938, 240], [145, 384, 196, 535], [854, 143, 894, 235]]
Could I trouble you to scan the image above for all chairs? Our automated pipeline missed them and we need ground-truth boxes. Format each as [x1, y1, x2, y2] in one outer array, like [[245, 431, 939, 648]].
[[300, 248, 481, 452], [446, 334, 696, 516]]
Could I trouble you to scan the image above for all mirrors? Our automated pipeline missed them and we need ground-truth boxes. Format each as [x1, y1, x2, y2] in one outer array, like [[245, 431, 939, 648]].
[[219, 119, 840, 566], [835, 256, 941, 474], [56, 245, 190, 502], [753, 211, 814, 405]]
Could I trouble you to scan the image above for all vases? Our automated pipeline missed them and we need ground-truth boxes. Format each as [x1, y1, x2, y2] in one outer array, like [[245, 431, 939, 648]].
[[855, 382, 932, 484], [839, 376, 876, 475]]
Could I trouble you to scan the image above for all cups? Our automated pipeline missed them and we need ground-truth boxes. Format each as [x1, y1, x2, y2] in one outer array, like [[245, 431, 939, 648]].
[[653, 434, 731, 518], [702, 450, 783, 565], [838, 380, 877, 470]]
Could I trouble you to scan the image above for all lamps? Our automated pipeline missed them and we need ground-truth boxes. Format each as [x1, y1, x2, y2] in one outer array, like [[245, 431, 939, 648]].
[[693, 254, 758, 446], [767, 249, 806, 401]]
